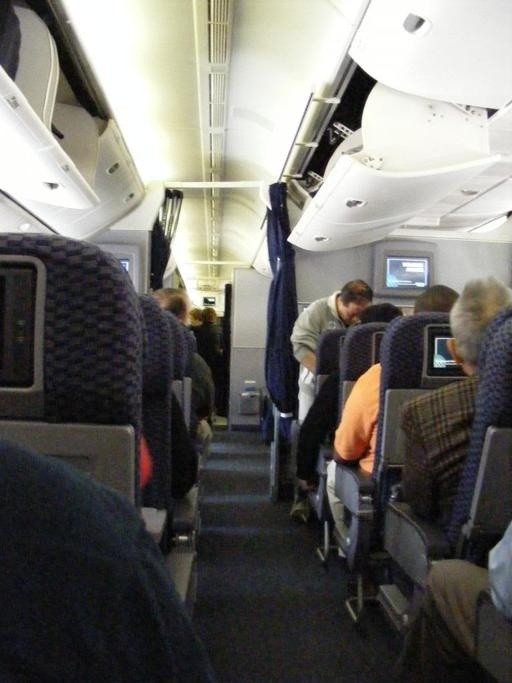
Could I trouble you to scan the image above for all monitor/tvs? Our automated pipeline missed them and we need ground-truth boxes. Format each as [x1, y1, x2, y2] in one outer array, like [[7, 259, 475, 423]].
[[0, 254, 47, 419], [203, 297, 216, 306], [372, 331, 386, 365], [421, 324, 471, 389], [109, 253, 134, 286], [376, 249, 434, 299]]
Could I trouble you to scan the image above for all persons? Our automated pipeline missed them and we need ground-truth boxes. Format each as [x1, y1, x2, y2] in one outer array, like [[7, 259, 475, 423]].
[[158, 293, 214, 460], [324, 283, 459, 560], [287, 278, 373, 524], [391, 518, 511, 683], [192, 307, 223, 407], [398, 276, 512, 525], [188, 307, 203, 329], [1, 439, 219, 682], [295, 302, 401, 490], [170, 393, 201, 502]]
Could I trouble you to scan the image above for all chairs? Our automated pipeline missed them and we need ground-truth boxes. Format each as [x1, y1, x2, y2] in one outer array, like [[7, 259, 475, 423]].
[[1, 232, 196, 621], [290, 307, 511, 683]]
